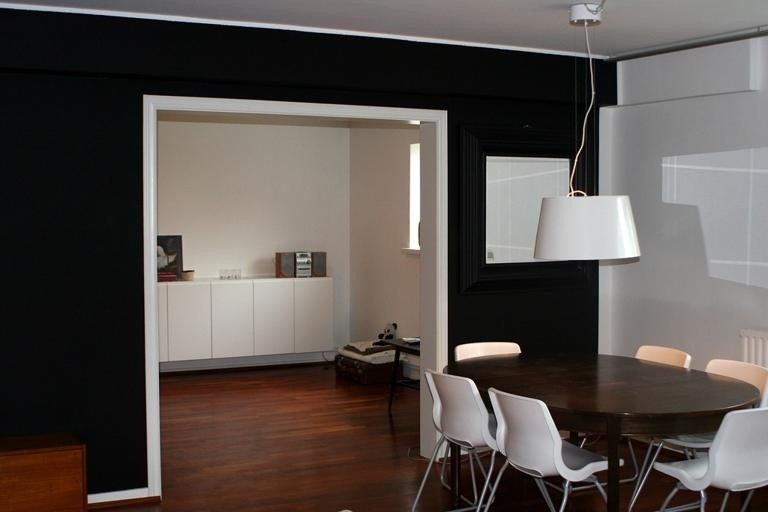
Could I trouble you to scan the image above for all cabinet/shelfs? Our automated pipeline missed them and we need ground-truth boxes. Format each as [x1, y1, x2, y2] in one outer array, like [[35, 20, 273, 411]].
[[387, 334, 421, 409], [158, 279, 335, 366]]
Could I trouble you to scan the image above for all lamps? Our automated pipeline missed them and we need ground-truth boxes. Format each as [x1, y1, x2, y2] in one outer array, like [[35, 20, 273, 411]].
[[533, 4, 642, 261]]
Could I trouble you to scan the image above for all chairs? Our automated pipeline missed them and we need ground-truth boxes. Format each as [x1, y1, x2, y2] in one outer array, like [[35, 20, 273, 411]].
[[651, 407, 768, 509], [412, 370, 549, 511], [441, 342, 584, 506], [628, 359, 768, 509], [482, 388, 624, 511], [620, 346, 692, 473]]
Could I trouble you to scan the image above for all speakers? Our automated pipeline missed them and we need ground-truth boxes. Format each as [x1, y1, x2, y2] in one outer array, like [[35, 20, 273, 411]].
[[311, 252, 326, 277], [276, 253, 295, 278]]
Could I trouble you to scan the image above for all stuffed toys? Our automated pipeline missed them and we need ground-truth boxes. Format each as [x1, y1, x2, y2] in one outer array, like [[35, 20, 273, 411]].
[[371, 322, 398, 346]]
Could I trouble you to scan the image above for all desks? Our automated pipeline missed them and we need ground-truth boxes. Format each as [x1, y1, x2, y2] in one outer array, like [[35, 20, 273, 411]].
[[443, 352, 761, 510]]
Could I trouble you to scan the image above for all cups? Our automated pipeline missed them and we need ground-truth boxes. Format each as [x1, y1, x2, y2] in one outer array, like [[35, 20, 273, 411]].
[[218, 269, 241, 280], [182, 270, 195, 280]]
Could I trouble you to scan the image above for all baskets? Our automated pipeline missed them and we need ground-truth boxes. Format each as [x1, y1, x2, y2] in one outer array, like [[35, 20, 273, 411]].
[[334, 355, 404, 386]]
